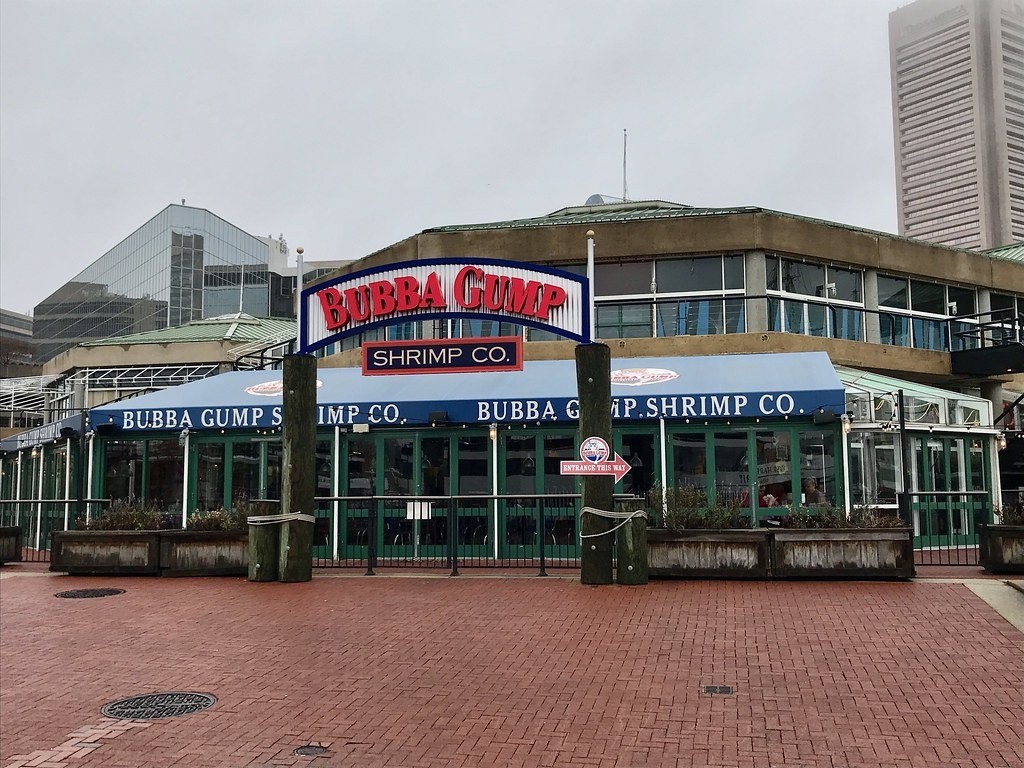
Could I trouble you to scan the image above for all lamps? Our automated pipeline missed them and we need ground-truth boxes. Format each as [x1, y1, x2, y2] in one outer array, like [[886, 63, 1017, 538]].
[[947, 301, 958, 315], [827, 281, 838, 297], [650, 277, 658, 292]]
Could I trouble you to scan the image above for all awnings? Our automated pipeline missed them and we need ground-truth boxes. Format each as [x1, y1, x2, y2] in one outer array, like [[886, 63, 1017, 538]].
[[88, 348, 848, 430]]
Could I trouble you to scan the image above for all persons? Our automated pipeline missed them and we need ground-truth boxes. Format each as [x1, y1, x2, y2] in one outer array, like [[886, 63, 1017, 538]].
[[743, 479, 783, 526], [804, 477, 825, 507]]
